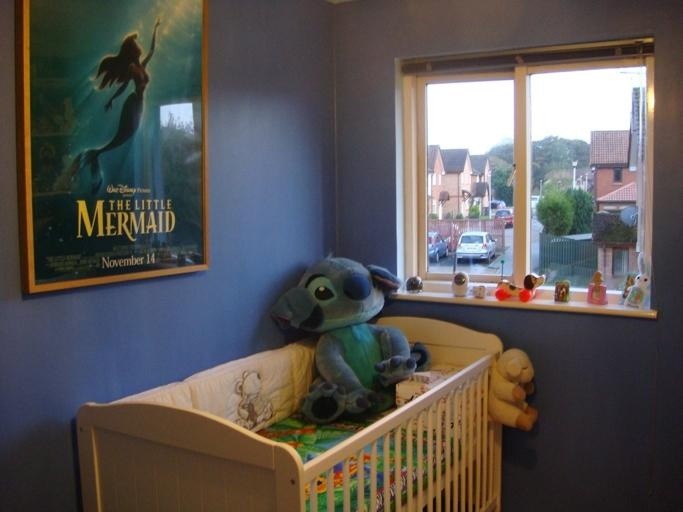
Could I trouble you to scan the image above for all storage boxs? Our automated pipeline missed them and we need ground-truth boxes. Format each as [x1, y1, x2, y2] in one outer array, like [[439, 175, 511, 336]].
[[387, 365, 483, 438]]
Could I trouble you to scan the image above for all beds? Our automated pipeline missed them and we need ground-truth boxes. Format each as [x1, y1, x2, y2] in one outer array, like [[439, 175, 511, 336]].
[[77, 316, 506, 512]]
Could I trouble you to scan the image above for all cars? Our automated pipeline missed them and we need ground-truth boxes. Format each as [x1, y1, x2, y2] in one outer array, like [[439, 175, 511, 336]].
[[494, 209, 513, 228], [427, 231, 449, 263], [455, 231, 497, 264]]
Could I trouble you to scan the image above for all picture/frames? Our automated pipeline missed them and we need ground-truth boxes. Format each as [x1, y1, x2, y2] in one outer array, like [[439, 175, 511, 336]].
[[14, 0, 212, 299]]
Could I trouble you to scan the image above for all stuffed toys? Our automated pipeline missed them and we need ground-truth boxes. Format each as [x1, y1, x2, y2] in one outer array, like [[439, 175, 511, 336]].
[[269, 249, 431, 424], [488, 341, 542, 438]]
